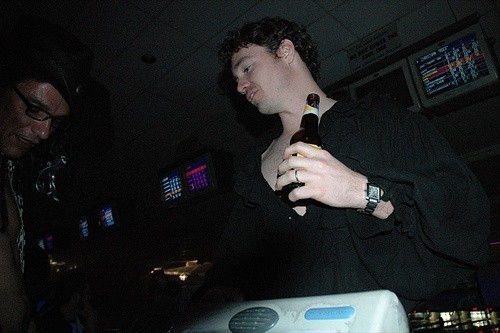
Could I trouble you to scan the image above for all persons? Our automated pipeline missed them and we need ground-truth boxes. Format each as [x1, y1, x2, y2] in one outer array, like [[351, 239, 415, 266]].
[[180, 17, 488, 329], [1, 18, 78, 333]]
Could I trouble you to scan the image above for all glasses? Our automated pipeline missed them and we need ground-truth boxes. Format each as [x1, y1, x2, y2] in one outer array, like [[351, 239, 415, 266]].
[[11, 86, 72, 132]]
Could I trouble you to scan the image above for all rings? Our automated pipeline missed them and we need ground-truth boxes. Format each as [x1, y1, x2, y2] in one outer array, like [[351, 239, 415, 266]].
[[295, 170, 300, 183]]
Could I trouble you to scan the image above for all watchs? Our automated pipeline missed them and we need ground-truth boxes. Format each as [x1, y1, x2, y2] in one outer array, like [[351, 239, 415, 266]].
[[358, 182, 381, 215]]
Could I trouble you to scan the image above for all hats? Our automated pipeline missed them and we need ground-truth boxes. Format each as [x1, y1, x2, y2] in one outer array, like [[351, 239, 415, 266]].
[[0, 14, 93, 121]]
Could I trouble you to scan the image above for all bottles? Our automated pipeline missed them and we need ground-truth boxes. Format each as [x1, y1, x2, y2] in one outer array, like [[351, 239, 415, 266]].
[[281, 94, 322, 201]]
